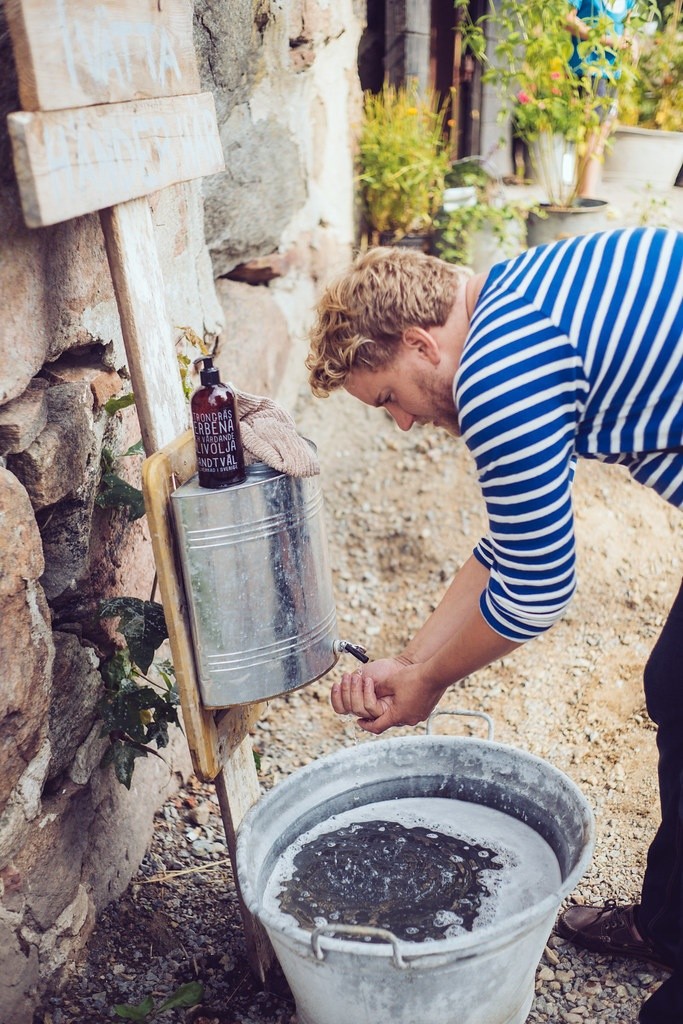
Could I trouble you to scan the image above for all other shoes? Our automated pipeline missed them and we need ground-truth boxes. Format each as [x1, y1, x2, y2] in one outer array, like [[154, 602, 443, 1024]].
[[557, 899, 676, 974]]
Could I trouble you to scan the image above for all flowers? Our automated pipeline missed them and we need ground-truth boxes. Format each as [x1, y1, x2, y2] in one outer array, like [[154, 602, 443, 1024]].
[[455, 0, 661, 209]]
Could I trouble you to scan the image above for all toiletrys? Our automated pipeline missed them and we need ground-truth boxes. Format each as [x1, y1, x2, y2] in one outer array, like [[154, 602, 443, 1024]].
[[190, 355, 248, 490]]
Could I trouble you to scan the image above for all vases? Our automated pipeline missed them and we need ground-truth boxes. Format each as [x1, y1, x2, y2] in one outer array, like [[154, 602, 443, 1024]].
[[526, 197, 607, 248]]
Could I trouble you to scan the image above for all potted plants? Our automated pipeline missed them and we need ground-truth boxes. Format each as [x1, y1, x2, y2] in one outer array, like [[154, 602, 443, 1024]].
[[351, 0, 683, 274]]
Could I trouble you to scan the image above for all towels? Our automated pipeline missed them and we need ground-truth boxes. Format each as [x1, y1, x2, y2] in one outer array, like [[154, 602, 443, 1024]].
[[224, 381, 323, 478]]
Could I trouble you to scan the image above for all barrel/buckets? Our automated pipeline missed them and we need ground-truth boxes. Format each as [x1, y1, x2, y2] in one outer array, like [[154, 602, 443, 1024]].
[[233, 710, 594, 1024]]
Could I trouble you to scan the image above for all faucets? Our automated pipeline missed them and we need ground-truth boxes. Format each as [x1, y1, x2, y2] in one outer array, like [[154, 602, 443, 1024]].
[[333, 639, 371, 665]]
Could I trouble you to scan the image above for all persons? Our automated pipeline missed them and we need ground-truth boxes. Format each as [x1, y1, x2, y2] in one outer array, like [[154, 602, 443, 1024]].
[[562, 0, 641, 200], [304, 228, 683, 1024]]
